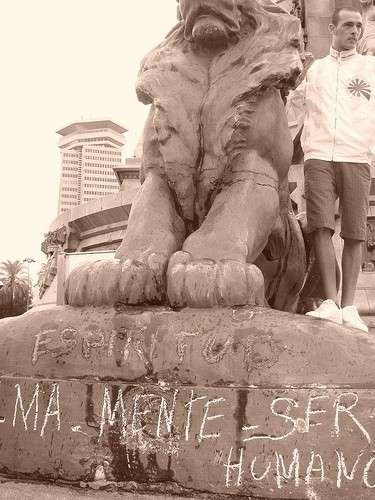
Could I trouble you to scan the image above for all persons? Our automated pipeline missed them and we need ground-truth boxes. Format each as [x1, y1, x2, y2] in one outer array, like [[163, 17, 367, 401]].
[[285, 7, 375, 332]]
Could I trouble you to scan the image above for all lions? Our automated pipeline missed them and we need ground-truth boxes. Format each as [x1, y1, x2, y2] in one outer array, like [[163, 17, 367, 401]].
[[62, 0, 341, 315]]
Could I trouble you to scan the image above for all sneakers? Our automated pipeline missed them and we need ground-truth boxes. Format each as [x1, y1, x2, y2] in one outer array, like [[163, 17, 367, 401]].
[[304, 299, 342, 324], [342, 305, 368, 333]]
